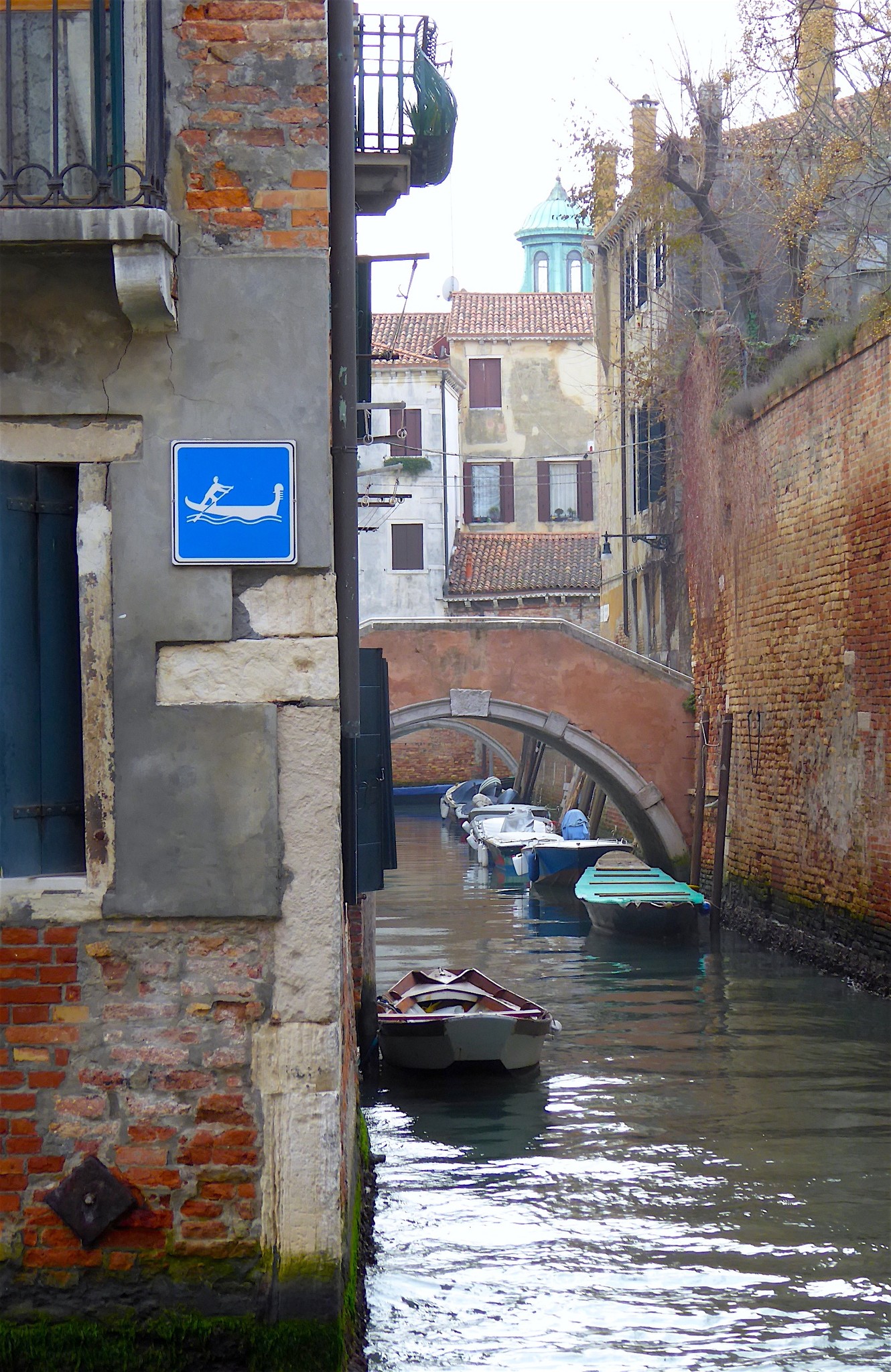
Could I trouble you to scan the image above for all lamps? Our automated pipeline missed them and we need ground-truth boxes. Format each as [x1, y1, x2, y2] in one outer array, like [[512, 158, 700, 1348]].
[[601, 531, 672, 561]]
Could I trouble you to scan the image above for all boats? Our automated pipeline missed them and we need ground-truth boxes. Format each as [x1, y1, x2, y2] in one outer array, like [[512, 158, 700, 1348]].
[[377, 963, 559, 1075], [525, 805, 634, 887], [574, 849, 709, 938], [456, 798, 563, 870], [438, 773, 518, 823]]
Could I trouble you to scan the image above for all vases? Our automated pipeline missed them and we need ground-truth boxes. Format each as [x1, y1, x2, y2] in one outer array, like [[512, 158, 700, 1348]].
[[473, 515, 488, 523]]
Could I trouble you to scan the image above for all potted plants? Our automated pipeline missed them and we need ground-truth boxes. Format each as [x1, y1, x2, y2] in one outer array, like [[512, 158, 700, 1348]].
[[489, 505, 501, 523], [554, 508, 565, 522], [566, 507, 577, 521]]
